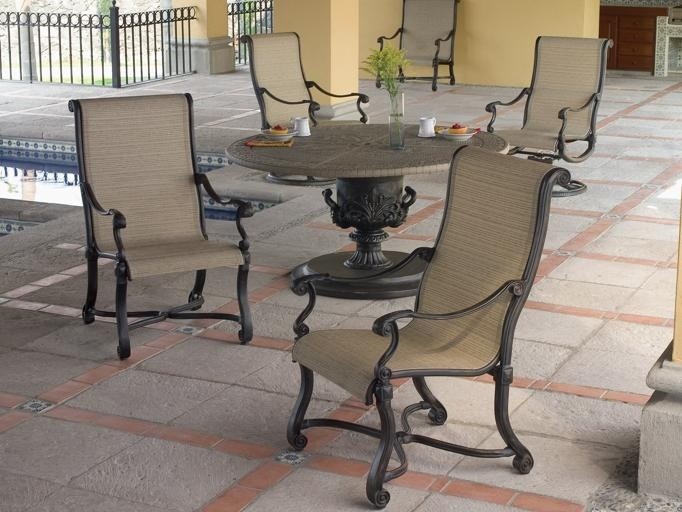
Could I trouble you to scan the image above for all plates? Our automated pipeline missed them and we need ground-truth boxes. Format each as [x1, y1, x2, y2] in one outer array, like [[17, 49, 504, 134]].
[[260, 128, 300, 143], [437, 129, 478, 142]]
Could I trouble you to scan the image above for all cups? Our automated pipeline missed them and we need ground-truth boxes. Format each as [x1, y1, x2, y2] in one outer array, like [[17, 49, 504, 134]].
[[292, 116, 312, 137], [417, 116, 437, 138]]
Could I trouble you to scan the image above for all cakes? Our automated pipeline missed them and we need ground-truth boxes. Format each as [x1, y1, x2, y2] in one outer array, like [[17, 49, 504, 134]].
[[447, 123, 468, 135], [270, 124, 288, 135]]
